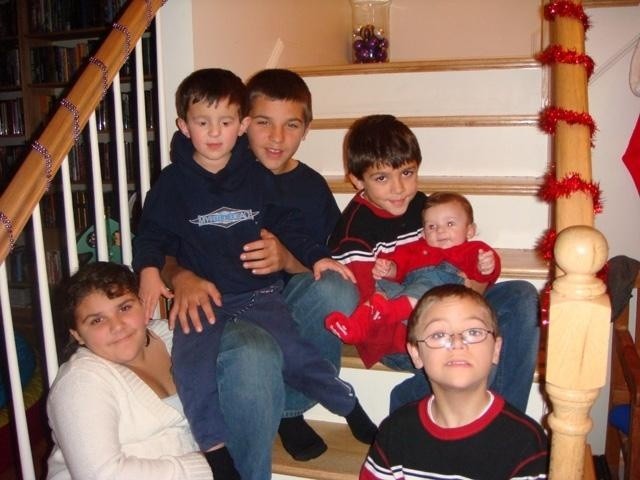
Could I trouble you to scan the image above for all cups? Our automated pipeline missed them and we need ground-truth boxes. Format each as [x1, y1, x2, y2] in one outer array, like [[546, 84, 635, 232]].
[[347, 0, 391, 65]]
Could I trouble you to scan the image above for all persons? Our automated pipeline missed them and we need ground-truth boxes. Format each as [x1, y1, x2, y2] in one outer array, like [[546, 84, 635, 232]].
[[328, 115, 541, 415], [357, 286, 547, 479], [45, 265, 215, 478], [127, 67, 379, 480], [322, 191, 503, 346], [159, 67, 357, 480]]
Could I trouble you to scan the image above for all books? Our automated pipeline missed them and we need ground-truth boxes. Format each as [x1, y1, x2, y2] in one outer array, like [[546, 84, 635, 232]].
[[1, 0, 157, 283]]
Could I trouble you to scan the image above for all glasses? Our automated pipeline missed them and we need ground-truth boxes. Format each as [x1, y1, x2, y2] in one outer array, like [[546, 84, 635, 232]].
[[416, 328, 497, 349]]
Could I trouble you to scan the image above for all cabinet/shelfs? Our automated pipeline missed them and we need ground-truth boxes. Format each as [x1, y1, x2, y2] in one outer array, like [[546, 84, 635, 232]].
[[0, 0, 154, 312]]
